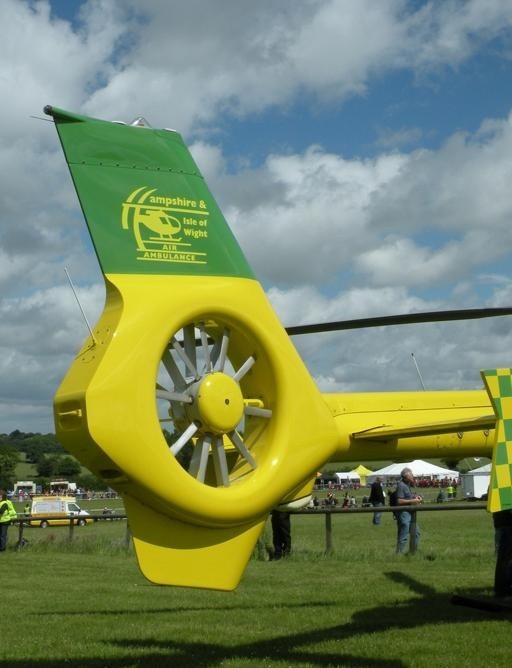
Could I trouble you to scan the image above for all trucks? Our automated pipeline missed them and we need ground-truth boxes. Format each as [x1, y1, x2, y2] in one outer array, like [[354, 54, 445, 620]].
[[30, 496, 94, 529]]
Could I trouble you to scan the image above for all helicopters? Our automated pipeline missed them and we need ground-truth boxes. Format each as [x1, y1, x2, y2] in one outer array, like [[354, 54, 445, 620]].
[[32, 94, 512, 596]]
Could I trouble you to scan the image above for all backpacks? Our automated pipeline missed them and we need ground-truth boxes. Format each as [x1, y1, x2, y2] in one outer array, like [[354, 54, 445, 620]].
[[389, 489, 400, 520]]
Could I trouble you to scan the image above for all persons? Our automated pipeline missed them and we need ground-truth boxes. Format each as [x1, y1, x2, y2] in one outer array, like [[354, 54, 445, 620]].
[[369, 475, 386, 524], [438, 489, 445, 502], [447, 484, 454, 497], [1, 488, 19, 551], [270, 509, 292, 559], [389, 466, 422, 552], [23, 502, 31, 526], [491, 509, 511, 598], [18, 488, 97, 501], [409, 475, 463, 488], [314, 478, 372, 509]]
